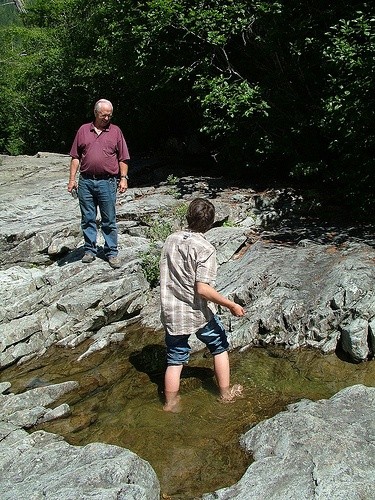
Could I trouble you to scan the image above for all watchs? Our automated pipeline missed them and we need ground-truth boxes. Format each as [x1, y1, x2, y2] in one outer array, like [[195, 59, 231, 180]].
[[120, 175, 129, 180]]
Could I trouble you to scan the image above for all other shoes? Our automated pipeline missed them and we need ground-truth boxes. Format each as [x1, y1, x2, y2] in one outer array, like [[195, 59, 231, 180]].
[[109, 257, 120, 268], [82, 254, 96, 263]]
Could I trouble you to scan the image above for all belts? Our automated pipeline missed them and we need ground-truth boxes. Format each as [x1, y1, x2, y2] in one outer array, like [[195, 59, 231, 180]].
[[83, 173, 115, 179]]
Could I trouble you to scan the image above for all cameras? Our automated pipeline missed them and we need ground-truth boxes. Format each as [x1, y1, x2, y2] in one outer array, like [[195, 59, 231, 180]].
[[72, 189, 78, 199]]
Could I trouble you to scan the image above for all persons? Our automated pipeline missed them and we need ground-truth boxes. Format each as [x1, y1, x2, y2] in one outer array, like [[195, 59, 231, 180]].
[[67, 98, 131, 268], [159, 197, 245, 413]]
